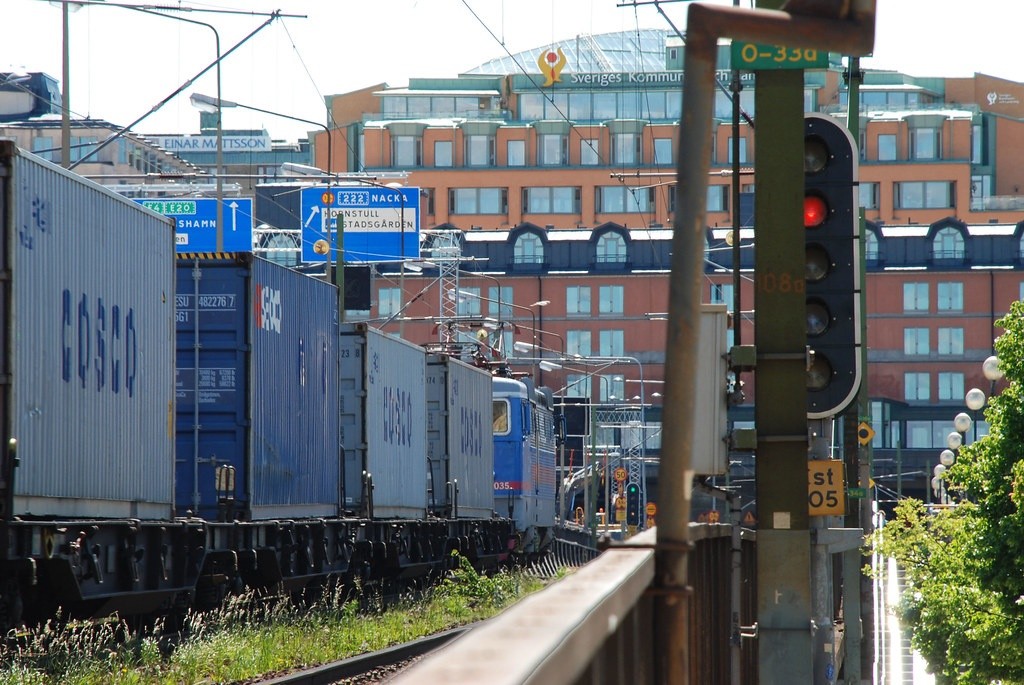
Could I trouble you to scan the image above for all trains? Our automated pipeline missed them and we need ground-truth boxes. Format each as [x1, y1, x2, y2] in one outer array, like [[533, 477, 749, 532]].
[[1, 142, 568, 568]]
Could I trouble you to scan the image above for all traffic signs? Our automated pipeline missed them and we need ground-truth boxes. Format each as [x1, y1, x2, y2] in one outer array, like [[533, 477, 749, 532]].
[[130, 197, 256, 259], [296, 186, 422, 264]]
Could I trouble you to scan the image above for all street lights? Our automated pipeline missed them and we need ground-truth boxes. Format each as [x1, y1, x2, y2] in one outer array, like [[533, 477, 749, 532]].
[[450, 287, 537, 388], [191, 93, 333, 281], [510, 340, 648, 531], [52, 0, 226, 254], [537, 360, 612, 531], [931, 412, 971, 505], [982, 355, 1006, 399], [966, 387, 987, 445]]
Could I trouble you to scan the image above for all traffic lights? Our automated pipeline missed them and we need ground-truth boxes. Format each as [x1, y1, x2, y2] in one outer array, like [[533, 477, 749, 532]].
[[626, 483, 641, 526], [795, 113, 866, 418]]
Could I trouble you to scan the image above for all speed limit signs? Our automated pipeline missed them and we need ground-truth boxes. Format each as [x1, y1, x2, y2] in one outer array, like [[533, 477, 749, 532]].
[[615, 468, 627, 481]]
